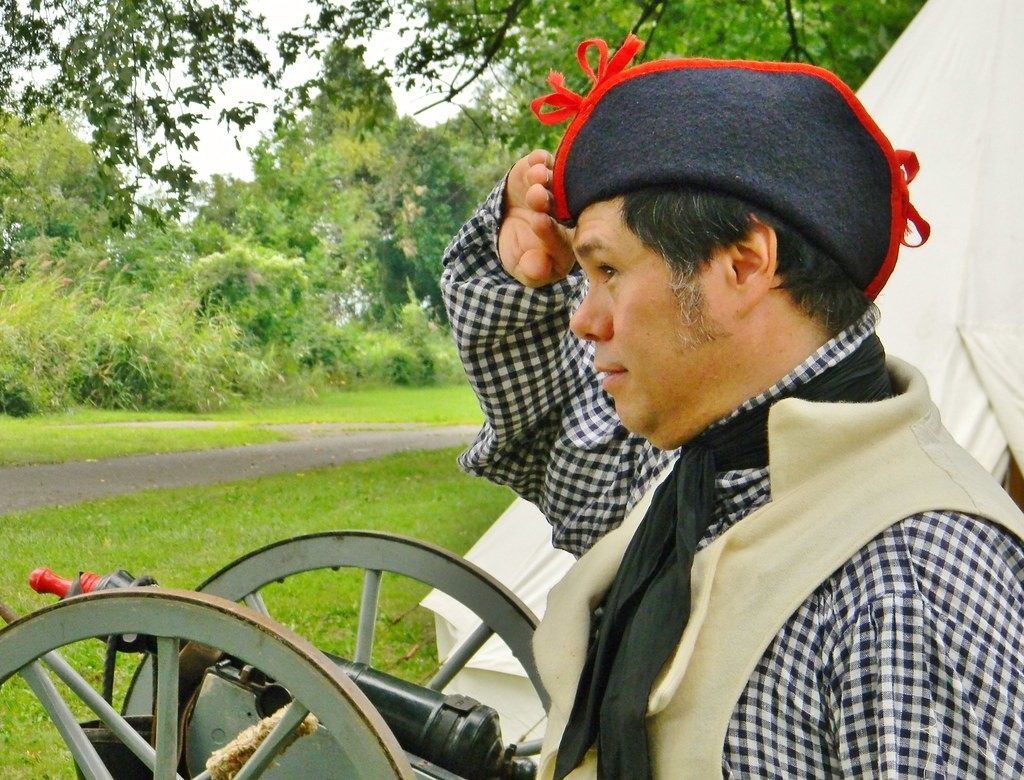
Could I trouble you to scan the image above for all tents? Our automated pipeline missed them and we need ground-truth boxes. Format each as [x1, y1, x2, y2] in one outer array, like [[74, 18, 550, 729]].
[[414, 0, 1024, 767]]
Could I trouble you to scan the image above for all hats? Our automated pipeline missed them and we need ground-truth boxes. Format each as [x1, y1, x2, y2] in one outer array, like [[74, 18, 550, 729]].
[[533, 32, 935, 303]]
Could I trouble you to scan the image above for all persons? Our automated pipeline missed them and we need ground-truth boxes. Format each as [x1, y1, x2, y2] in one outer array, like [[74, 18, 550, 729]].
[[441, 34, 1022, 780]]
